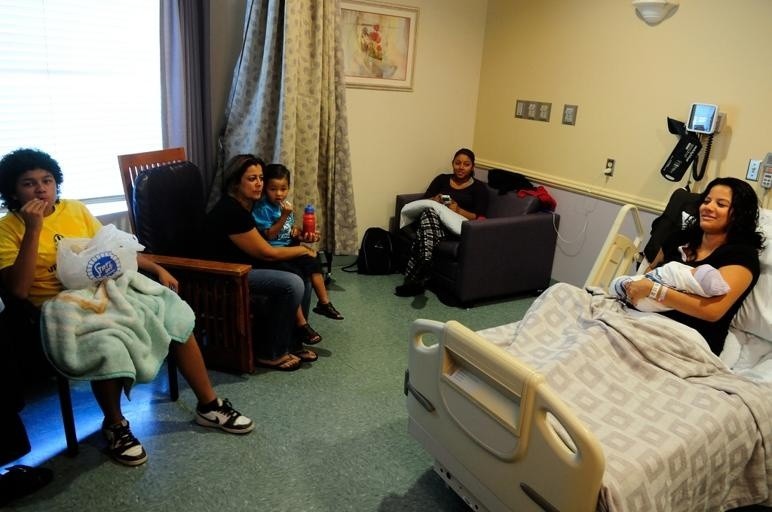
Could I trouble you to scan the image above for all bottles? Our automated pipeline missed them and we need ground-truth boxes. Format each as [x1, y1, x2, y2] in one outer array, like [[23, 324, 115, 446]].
[[301, 203, 317, 238]]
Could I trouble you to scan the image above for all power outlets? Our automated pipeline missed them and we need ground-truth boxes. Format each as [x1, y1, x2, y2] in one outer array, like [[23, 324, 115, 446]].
[[604, 159, 615, 176]]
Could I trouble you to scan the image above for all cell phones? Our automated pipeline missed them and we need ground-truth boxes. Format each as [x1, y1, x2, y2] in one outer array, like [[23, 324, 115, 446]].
[[440, 194, 450, 201]]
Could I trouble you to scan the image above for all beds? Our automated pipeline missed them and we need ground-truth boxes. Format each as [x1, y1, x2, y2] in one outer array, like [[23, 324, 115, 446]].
[[403, 202, 772, 512]]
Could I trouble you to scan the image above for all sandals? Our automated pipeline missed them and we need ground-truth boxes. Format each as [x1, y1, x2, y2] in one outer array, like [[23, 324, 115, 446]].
[[295, 322, 323, 346], [311, 299, 345, 322]]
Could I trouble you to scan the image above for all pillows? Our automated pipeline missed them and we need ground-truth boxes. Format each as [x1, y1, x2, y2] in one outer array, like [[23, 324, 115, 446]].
[[485, 183, 541, 218], [683, 207, 772, 344], [133, 159, 207, 255]]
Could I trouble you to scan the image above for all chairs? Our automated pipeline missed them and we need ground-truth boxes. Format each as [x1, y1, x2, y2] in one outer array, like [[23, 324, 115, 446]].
[[391, 192, 560, 310], [0, 267, 179, 457], [116, 146, 252, 374]]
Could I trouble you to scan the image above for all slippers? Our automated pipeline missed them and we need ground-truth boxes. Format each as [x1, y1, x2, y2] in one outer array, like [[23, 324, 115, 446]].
[[258, 352, 301, 372], [285, 344, 319, 362]]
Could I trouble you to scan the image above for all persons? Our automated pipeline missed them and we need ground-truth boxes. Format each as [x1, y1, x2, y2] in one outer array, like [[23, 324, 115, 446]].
[[394, 146, 491, 299], [624, 175, 763, 362], [611, 259, 731, 313], [0, 147, 256, 468], [252, 164, 343, 344], [200, 149, 320, 371]]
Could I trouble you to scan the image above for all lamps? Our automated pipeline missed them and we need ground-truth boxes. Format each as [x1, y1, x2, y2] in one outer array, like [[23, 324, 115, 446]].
[[632, 0, 679, 27]]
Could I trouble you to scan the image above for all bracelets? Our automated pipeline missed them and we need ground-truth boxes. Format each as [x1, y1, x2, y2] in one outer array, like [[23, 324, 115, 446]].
[[656, 286, 668, 304], [648, 281, 661, 301]]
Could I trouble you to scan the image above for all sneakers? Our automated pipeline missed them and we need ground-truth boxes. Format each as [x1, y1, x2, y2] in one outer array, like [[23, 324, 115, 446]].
[[192, 396, 256, 437], [100, 414, 149, 468], [393, 276, 427, 298]]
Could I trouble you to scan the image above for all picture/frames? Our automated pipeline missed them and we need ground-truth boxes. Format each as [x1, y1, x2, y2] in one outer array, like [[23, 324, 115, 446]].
[[337, 1, 421, 92]]
